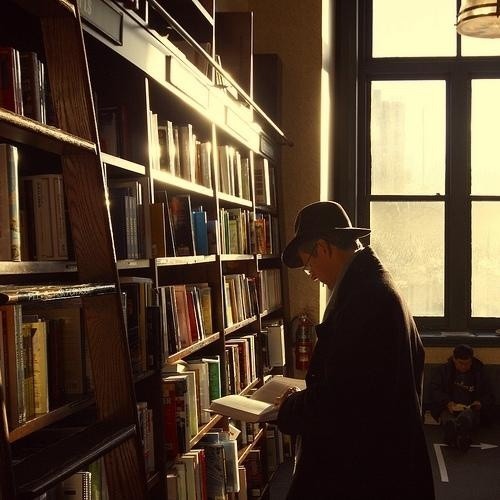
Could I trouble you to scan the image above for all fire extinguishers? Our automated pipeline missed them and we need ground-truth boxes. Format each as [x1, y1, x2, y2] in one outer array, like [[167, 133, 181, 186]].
[[290, 312, 316, 371]]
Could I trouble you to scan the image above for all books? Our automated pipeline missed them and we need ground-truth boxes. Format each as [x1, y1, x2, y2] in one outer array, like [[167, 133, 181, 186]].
[[198, 376, 307, 424], [1, 268, 292, 499], [450, 403, 479, 412], [2, 37, 276, 263]]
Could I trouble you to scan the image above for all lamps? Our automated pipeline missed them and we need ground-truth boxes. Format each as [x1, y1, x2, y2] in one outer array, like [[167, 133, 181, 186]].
[[457, 0, 500, 38]]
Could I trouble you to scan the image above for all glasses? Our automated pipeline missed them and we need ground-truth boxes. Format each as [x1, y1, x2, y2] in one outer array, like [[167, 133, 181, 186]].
[[302, 244, 317, 276]]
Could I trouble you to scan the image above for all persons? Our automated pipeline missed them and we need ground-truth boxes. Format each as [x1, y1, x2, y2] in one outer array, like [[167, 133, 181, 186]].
[[274, 201, 436, 499], [429, 344, 497, 471]]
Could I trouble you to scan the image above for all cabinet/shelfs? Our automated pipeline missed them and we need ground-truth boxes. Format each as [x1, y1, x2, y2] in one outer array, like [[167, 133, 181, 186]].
[[0, 0, 297, 500]]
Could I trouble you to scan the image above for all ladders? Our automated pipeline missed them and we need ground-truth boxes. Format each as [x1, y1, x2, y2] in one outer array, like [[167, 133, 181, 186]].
[[0, 0, 150, 500]]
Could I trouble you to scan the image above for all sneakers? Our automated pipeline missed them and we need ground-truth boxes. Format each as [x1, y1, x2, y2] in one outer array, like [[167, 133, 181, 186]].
[[447, 437, 466, 450]]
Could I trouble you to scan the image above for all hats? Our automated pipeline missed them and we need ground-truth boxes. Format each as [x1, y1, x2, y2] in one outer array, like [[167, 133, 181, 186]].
[[281, 201, 371, 268]]
